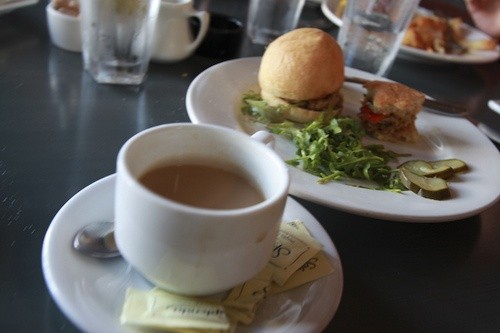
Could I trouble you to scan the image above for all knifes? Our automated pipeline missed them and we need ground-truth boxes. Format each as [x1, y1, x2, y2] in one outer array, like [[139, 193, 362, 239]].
[[343, 75, 470, 117]]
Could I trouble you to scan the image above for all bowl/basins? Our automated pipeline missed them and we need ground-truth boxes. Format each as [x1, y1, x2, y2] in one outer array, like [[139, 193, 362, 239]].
[[46, 0, 82, 52]]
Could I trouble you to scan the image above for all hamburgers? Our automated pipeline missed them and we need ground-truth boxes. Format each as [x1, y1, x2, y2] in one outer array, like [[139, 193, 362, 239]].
[[257, 28, 344, 124]]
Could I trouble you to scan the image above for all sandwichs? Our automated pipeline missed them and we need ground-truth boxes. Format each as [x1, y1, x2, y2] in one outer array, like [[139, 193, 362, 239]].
[[359, 82, 424, 144]]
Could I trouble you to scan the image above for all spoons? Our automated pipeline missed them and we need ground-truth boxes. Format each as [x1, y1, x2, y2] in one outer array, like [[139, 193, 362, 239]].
[[74, 221, 123, 258]]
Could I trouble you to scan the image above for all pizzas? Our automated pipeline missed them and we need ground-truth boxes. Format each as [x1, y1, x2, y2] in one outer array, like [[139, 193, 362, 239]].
[[335, 0, 496, 55]]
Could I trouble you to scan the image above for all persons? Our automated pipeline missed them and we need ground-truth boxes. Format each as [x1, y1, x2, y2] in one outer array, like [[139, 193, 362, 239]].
[[466, 0, 500, 39]]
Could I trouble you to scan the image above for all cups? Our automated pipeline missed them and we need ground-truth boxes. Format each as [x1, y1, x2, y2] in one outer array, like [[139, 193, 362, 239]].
[[336, 0, 419, 74], [114, 122, 290, 297], [80, 0, 161, 85], [246, 0, 306, 46], [151, 0, 210, 61]]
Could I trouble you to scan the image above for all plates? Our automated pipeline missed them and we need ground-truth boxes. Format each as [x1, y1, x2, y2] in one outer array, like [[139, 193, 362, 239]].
[[40, 172, 344, 332], [184, 55, 500, 223], [321, 1, 500, 63]]
